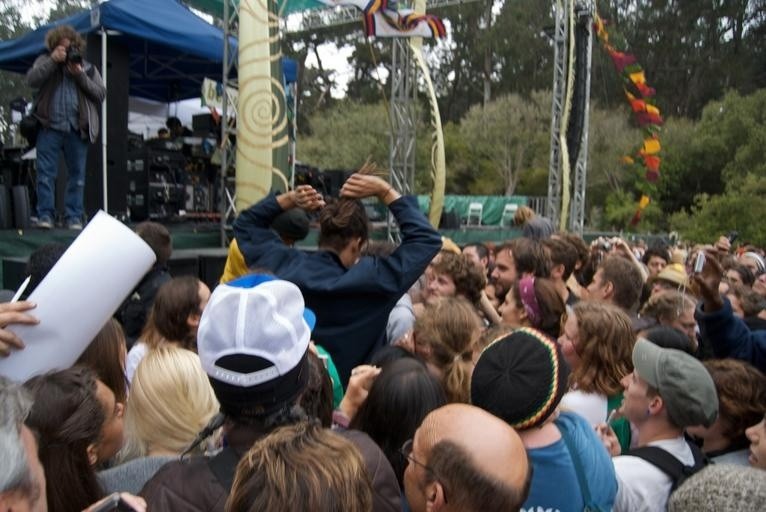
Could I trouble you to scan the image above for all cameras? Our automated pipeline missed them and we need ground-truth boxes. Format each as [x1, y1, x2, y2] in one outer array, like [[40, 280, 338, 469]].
[[728, 232, 739, 244], [600, 240, 612, 251], [694, 251, 706, 273], [63, 42, 83, 64]]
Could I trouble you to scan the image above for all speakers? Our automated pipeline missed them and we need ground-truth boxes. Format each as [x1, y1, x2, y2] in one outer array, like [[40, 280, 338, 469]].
[[437, 208, 460, 229]]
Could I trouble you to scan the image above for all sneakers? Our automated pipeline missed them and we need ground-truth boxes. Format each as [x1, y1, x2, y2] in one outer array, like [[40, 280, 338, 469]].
[[63, 214, 84, 231], [33, 214, 55, 231]]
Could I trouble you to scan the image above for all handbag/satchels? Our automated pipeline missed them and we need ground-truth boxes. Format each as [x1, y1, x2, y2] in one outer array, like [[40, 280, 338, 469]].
[[19, 114, 42, 137]]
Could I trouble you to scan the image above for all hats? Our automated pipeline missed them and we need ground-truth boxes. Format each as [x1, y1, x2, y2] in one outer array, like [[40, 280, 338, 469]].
[[468, 325, 569, 429], [647, 262, 695, 290], [632, 336, 720, 430], [195, 272, 316, 388]]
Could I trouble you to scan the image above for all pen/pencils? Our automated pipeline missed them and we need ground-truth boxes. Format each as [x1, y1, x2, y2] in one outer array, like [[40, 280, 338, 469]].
[[605, 408, 619, 434], [11, 274, 32, 302]]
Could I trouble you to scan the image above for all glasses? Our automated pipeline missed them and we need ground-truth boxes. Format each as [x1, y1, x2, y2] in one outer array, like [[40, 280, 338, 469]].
[[398, 438, 454, 504]]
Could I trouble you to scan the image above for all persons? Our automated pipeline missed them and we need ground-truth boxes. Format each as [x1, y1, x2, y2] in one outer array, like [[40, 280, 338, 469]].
[[0, 171, 766, 512], [166, 117, 193, 157], [158, 128, 168, 138], [26, 22, 106, 229]]
